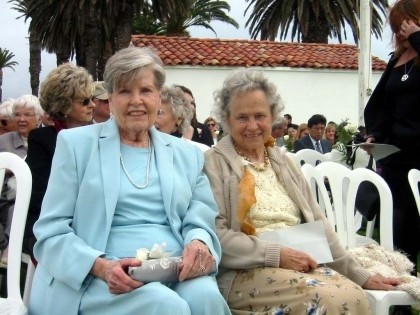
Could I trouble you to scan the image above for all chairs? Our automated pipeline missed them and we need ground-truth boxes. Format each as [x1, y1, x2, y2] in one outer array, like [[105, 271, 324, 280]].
[[0, 140, 420, 315]]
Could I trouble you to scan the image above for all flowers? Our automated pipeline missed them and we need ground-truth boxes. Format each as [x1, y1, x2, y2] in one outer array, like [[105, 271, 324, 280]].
[[214, 130, 225, 142], [283, 133, 294, 153], [332, 117, 361, 155]]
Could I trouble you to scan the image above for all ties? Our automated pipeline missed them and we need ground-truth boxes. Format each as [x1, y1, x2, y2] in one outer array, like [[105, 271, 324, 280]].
[[315, 141, 322, 154]]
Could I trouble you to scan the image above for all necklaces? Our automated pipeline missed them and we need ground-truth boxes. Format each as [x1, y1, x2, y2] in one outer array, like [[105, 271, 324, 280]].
[[401, 59, 416, 81], [120, 138, 150, 188]]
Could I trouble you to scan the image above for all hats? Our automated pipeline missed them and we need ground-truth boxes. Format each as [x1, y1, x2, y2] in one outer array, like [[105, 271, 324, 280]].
[[92, 80, 109, 100]]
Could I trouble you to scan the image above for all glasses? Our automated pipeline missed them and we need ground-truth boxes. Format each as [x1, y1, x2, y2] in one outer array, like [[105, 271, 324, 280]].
[[12, 111, 33, 120], [157, 109, 163, 118], [206, 123, 215, 127], [74, 95, 95, 106], [0, 119, 9, 126]]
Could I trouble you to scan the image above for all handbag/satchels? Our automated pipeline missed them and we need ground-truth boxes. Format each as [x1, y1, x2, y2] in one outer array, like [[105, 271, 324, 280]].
[[349, 164, 381, 221], [128, 257, 183, 286]]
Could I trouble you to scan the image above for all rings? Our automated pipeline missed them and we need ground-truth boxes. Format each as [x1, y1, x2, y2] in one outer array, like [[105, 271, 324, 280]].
[[200, 265, 205, 270]]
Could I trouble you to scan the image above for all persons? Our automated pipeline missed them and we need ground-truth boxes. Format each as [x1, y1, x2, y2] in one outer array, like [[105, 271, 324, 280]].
[[0, 94, 55, 270], [27, 47, 233, 315], [202, 69, 401, 315], [272, 114, 292, 146], [204, 118, 219, 146], [294, 114, 332, 164], [22, 63, 96, 254], [288, 123, 299, 140], [154, 86, 194, 138], [364, 0, 420, 276], [326, 121, 337, 146], [179, 85, 211, 147], [88, 81, 110, 124], [296, 123, 309, 139]]
